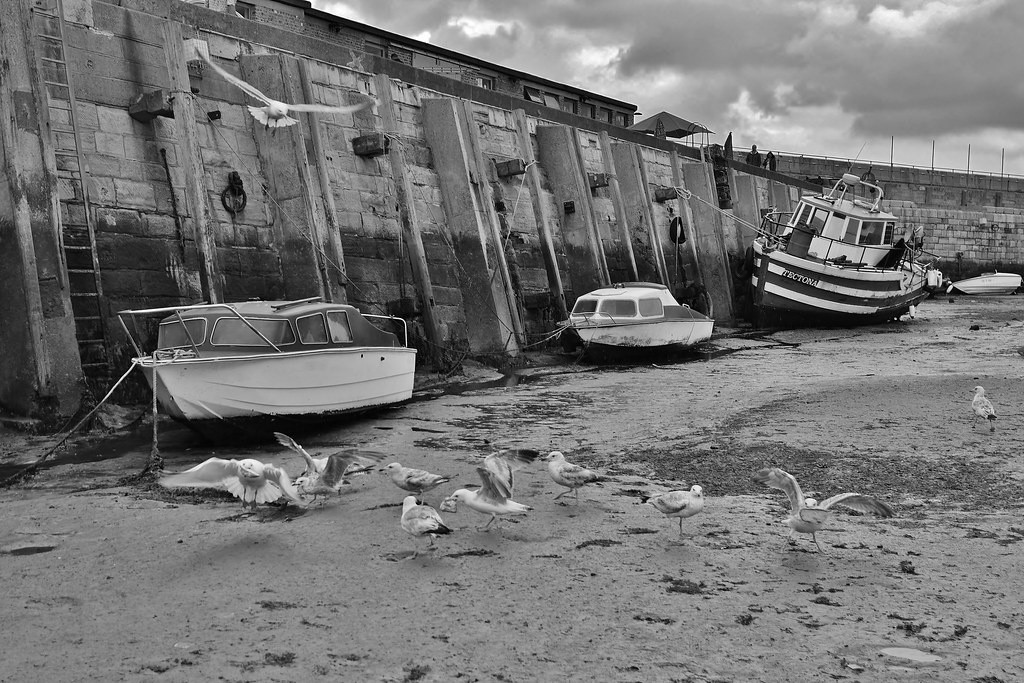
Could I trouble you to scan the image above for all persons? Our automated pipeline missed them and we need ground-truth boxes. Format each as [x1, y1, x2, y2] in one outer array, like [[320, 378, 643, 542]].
[[763, 151, 776, 171], [391, 53, 404, 63], [745, 145, 761, 167]]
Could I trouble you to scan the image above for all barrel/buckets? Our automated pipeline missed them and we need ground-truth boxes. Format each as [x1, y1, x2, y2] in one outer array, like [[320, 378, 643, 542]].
[[786, 223, 816, 258]]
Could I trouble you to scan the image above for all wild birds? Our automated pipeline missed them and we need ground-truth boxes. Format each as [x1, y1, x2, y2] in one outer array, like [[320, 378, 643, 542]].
[[193, 42, 372, 134], [150, 430, 895, 560], [971, 386, 998, 431]]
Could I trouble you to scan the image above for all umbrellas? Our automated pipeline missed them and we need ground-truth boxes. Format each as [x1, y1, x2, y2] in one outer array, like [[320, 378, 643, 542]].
[[625, 112, 716, 139]]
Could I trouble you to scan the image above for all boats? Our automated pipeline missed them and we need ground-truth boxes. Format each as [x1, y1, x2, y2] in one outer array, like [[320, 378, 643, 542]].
[[735, 175, 933, 331], [559, 282, 717, 360], [950, 269, 1022, 297], [115, 295, 418, 443]]
